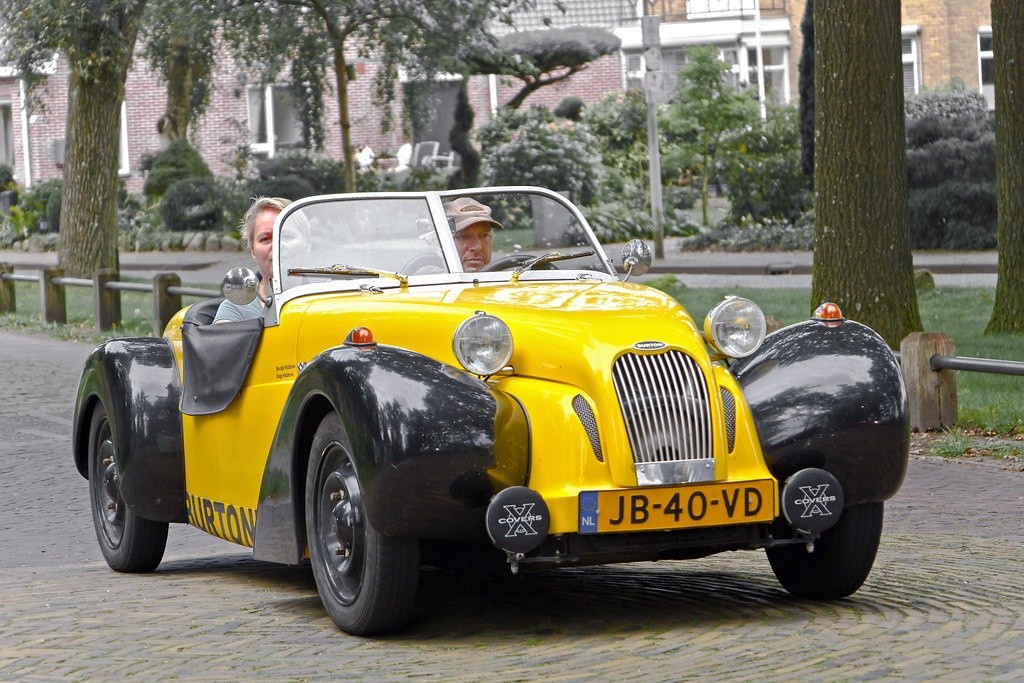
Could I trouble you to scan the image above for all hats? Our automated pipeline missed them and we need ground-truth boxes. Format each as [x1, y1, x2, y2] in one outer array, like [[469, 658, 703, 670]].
[[442, 196, 504, 237]]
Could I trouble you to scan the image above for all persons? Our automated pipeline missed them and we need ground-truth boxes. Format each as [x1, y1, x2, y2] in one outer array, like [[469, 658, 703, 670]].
[[443, 197, 503, 274], [211, 197, 312, 324], [355, 144, 375, 174]]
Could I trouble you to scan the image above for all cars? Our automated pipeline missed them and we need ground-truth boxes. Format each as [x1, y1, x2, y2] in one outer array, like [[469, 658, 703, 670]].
[[74, 185, 912, 641]]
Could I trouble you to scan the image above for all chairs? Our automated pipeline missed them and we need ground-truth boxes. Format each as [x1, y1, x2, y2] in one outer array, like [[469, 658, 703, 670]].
[[412, 141, 440, 167]]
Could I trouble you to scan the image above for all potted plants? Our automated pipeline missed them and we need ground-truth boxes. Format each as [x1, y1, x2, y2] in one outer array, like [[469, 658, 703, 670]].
[[376, 151, 398, 169]]
